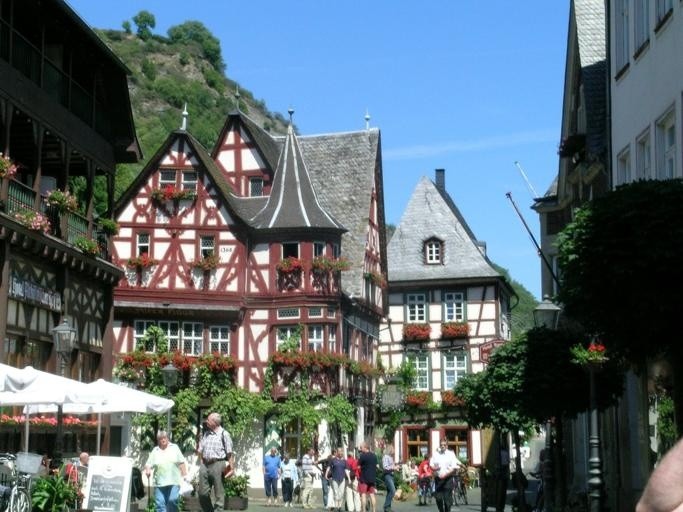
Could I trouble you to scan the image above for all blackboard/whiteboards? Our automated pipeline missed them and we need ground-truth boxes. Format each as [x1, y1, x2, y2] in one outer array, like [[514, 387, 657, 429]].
[[81, 456, 132, 512]]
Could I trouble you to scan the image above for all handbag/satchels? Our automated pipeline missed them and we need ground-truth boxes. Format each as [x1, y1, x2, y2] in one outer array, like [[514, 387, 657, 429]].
[[223, 464, 232, 478], [294, 484, 300, 495]]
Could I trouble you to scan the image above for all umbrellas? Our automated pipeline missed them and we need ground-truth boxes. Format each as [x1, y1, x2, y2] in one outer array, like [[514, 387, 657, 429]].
[[17, 364, 108, 454], [0, 363, 75, 405], [21, 378, 176, 455]]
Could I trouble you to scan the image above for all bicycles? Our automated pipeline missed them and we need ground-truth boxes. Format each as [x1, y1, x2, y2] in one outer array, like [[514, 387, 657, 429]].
[[420, 480, 431, 504], [452, 473, 468, 505]]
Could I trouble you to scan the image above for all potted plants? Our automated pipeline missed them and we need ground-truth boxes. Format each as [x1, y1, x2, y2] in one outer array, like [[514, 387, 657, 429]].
[[224, 473, 251, 511]]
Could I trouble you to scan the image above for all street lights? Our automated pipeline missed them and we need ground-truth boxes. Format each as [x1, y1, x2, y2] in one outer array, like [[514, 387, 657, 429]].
[[161, 360, 179, 442], [53, 319, 76, 457]]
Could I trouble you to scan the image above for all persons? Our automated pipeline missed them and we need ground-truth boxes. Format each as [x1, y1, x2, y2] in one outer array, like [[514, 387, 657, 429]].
[[142, 431, 189, 512], [78, 451, 90, 467], [259, 437, 468, 512], [194, 411, 235, 511]]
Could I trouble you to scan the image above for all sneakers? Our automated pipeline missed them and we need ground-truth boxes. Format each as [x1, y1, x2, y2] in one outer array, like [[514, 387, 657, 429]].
[[302, 502, 315, 509], [384, 506, 392, 511], [265, 500, 293, 507]]
